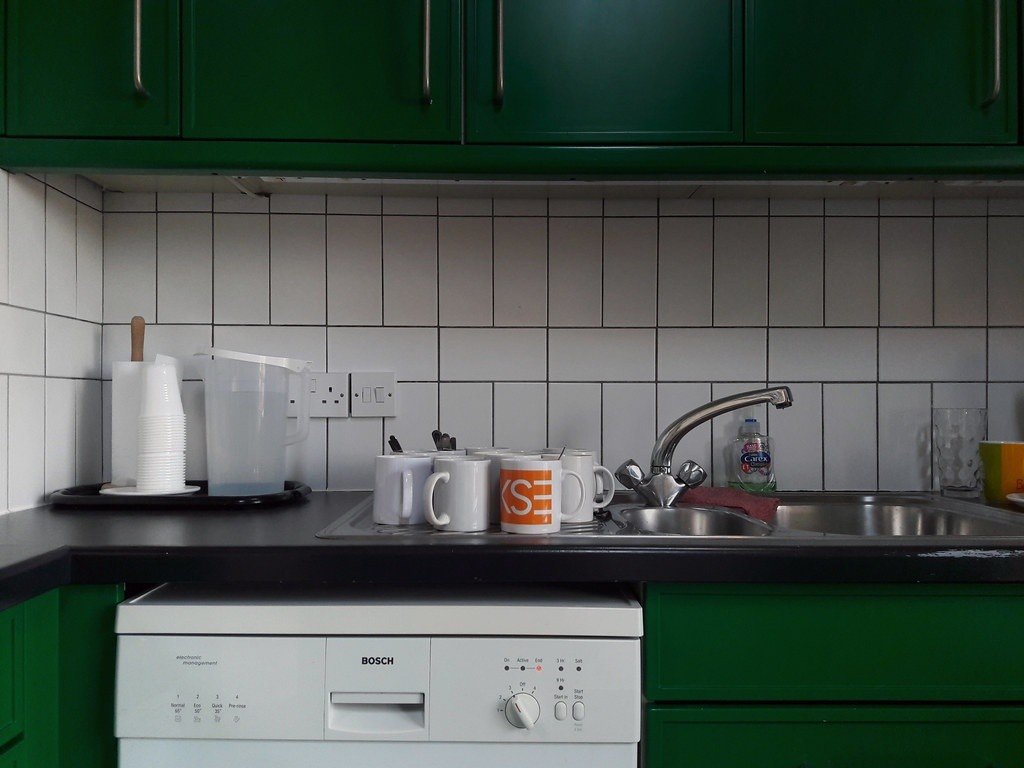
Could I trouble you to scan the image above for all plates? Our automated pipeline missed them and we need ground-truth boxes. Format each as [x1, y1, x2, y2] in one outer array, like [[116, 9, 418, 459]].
[[99, 486, 200, 495], [1006, 493, 1024, 502]]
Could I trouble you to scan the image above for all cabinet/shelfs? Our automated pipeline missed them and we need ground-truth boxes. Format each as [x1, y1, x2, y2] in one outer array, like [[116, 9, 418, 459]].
[[186, 2, 740, 174], [745, 0, 1024, 177], [0, 0, 179, 171], [0, 582, 1024, 768]]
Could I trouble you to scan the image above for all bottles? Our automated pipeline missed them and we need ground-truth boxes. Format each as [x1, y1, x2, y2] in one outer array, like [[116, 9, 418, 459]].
[[732, 419, 777, 491]]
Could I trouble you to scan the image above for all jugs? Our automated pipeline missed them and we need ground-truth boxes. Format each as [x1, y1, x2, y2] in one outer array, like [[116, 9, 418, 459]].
[[193, 348, 312, 495]]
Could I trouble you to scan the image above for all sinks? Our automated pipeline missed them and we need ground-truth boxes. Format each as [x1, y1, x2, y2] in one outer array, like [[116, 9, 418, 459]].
[[608, 503, 776, 536], [772, 492, 1022, 539]]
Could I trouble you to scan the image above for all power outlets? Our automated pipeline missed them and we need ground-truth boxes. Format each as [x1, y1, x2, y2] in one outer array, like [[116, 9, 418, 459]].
[[306, 371, 349, 419]]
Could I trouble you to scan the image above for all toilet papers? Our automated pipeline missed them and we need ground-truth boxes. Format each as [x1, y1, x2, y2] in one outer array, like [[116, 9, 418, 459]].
[[110, 360, 154, 486]]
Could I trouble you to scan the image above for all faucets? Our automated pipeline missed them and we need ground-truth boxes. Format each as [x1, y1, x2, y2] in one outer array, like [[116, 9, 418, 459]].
[[648, 386, 794, 476]]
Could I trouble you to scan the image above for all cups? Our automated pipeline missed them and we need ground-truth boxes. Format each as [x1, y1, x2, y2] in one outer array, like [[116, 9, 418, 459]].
[[137, 364, 186, 490], [373, 446, 615, 534], [980, 440, 1024, 501], [933, 407, 987, 498]]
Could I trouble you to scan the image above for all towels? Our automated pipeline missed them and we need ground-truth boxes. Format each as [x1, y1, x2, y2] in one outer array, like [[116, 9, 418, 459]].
[[674, 485, 781, 523]]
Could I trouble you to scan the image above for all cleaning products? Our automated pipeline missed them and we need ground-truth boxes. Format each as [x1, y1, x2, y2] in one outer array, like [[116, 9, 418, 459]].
[[727, 418, 777, 494]]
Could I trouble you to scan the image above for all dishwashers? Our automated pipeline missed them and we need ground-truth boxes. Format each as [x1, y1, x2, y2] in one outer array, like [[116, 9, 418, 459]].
[[114, 583, 644, 768]]
